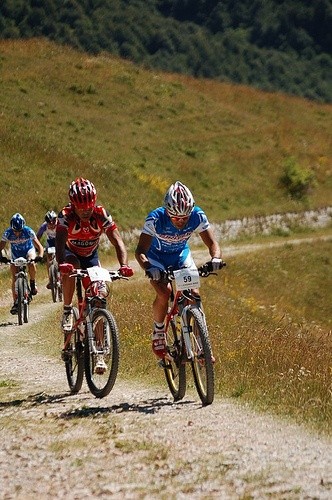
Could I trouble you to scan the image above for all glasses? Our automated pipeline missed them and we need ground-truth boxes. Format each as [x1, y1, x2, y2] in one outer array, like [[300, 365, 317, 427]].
[[12, 229, 23, 232], [73, 204, 94, 214], [167, 212, 191, 222], [47, 221, 56, 225]]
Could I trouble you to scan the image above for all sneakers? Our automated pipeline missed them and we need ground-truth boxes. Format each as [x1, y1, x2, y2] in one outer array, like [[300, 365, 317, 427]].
[[62, 311, 73, 334], [196, 347, 215, 366], [152, 331, 167, 357], [93, 355, 109, 373]]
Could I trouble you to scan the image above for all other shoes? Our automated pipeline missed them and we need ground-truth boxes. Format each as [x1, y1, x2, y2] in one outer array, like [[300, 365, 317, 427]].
[[47, 283, 51, 289], [10, 305, 19, 313], [30, 283, 37, 295]]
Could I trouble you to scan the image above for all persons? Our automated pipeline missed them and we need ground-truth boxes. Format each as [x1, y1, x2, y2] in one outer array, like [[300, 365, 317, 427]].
[[135, 181, 223, 364], [36, 211, 58, 289], [0, 213, 44, 314], [55, 178, 134, 375]]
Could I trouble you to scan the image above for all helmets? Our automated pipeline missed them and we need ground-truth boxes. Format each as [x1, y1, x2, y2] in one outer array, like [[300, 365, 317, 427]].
[[68, 177, 97, 209], [45, 210, 58, 222], [10, 213, 26, 231], [164, 181, 195, 215]]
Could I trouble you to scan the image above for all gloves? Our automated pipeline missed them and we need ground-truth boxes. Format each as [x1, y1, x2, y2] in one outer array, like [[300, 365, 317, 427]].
[[145, 267, 167, 281], [0, 257, 8, 264], [207, 258, 224, 272], [58, 261, 74, 274], [117, 266, 133, 277], [35, 257, 43, 263]]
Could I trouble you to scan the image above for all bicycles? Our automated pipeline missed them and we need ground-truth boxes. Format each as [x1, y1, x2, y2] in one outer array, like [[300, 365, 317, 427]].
[[35, 246, 65, 304], [0, 258, 38, 324], [146, 261, 228, 405], [57, 266, 134, 398]]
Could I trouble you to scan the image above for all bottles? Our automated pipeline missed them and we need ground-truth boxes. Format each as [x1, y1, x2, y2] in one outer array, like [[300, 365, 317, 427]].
[[78, 322, 85, 347]]
